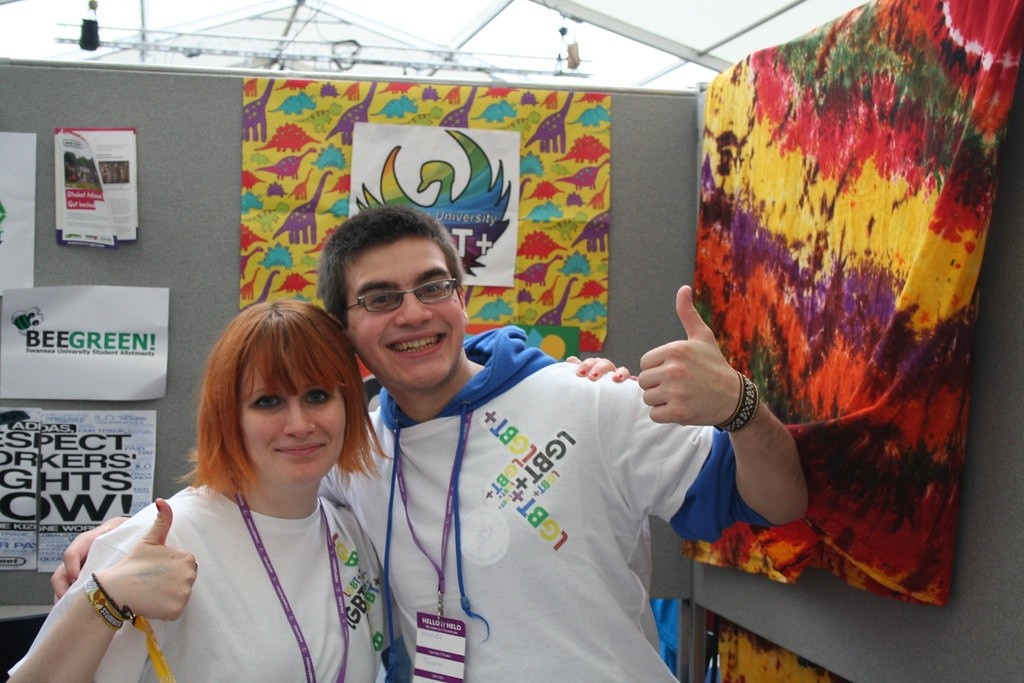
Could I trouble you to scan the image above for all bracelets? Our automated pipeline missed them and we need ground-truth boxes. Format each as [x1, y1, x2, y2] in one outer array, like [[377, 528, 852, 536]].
[[713, 370, 760, 433], [83, 573, 175, 683]]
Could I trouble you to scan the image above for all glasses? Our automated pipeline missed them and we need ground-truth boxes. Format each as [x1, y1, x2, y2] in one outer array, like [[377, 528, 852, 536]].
[[345, 278, 457, 312]]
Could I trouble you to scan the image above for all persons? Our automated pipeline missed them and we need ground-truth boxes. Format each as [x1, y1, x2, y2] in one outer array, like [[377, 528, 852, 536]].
[[51, 205, 809, 682], [1, 300, 640, 683]]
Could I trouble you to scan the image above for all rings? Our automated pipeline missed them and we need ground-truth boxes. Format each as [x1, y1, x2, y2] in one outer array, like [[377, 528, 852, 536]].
[[195, 561, 198, 571]]
